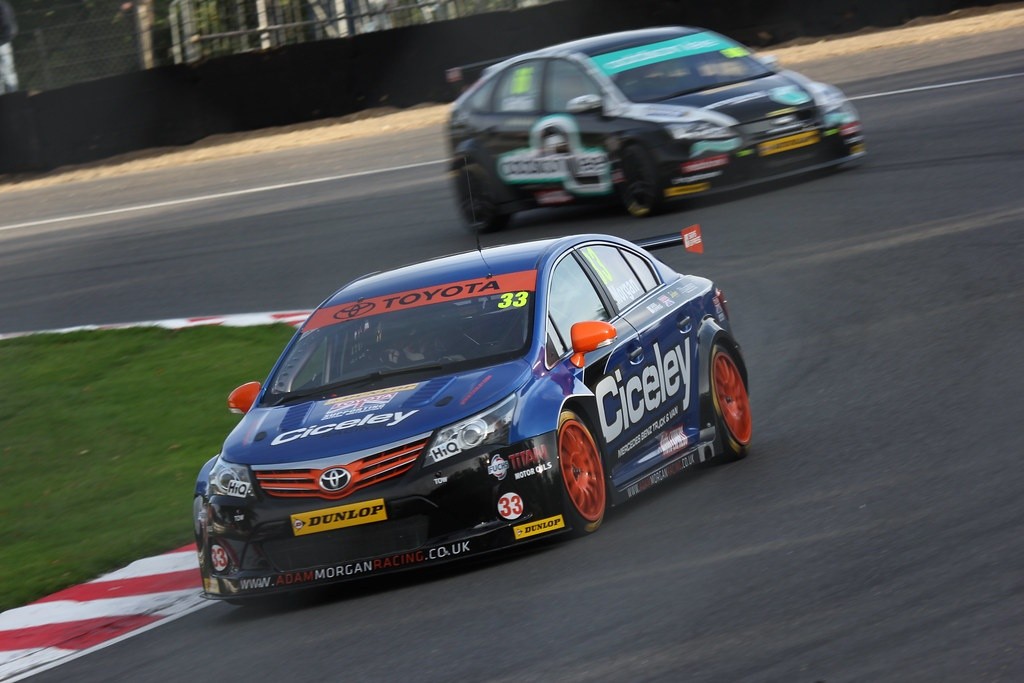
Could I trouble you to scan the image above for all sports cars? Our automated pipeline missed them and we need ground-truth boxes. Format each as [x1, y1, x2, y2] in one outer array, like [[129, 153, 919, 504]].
[[443, 25, 869, 233], [191, 222, 754, 613]]
